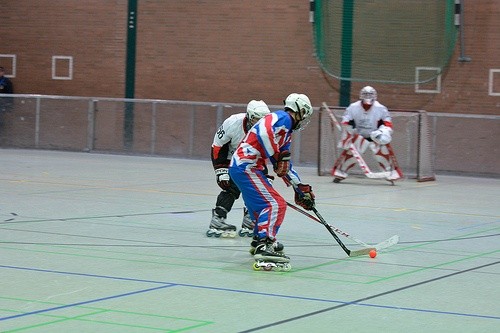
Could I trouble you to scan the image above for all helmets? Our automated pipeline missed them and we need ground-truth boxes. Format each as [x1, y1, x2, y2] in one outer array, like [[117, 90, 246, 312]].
[[285, 93, 313, 117], [246, 99, 270, 120], [359, 86, 377, 101]]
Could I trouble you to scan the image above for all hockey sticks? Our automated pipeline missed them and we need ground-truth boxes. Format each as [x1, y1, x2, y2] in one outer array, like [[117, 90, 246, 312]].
[[285, 172, 376, 257], [288, 202, 399, 252], [321, 101, 391, 179]]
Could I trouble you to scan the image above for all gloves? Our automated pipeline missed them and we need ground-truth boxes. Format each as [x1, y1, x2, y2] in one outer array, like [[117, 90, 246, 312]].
[[217, 173, 230, 189], [294, 184, 315, 209], [275, 152, 292, 176]]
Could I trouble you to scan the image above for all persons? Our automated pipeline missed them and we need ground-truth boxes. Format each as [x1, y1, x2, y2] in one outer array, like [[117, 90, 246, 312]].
[[206, 100, 270, 238], [0, 67, 13, 148], [228, 93, 316, 272], [331, 86, 404, 184]]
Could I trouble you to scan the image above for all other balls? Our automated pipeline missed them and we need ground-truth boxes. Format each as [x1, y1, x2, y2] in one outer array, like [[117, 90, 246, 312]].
[[369, 250, 376, 257]]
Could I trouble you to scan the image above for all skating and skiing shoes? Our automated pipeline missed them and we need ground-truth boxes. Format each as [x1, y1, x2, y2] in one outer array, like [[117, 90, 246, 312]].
[[249, 236, 291, 271], [239, 207, 255, 237], [250, 230, 284, 255], [206, 208, 237, 238]]
